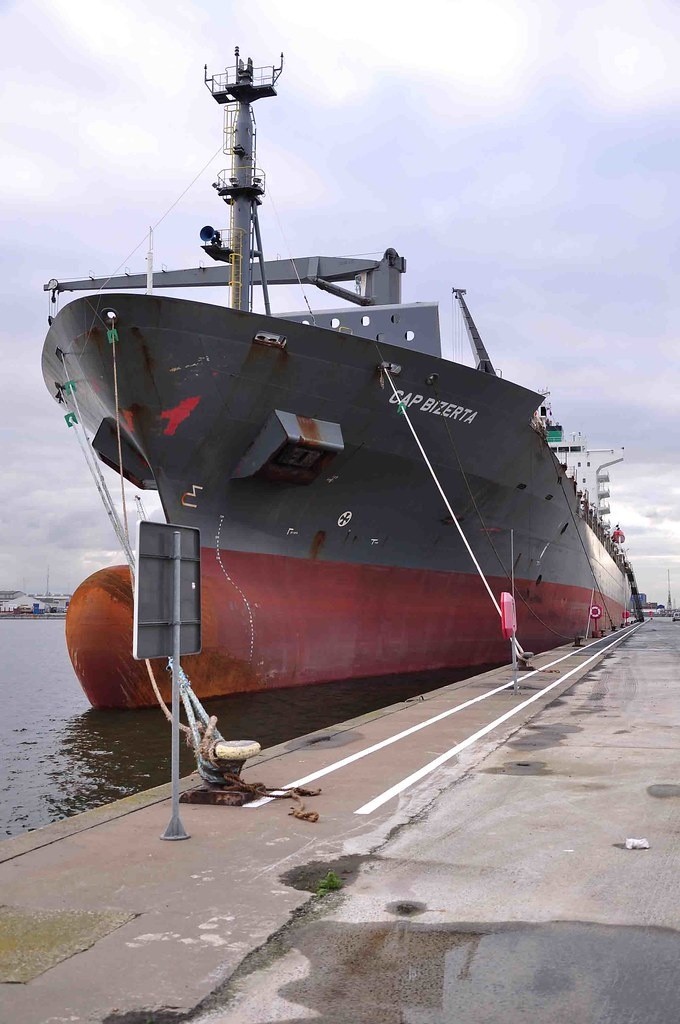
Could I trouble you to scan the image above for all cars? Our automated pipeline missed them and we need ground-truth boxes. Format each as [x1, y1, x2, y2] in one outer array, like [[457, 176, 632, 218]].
[[673, 612, 680, 621], [18, 604, 67, 615]]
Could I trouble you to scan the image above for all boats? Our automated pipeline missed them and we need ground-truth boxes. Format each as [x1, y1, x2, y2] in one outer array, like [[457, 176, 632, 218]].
[[40, 46, 644, 711]]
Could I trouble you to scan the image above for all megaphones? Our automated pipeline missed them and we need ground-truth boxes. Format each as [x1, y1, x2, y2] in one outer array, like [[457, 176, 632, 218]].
[[200, 226, 216, 241]]
